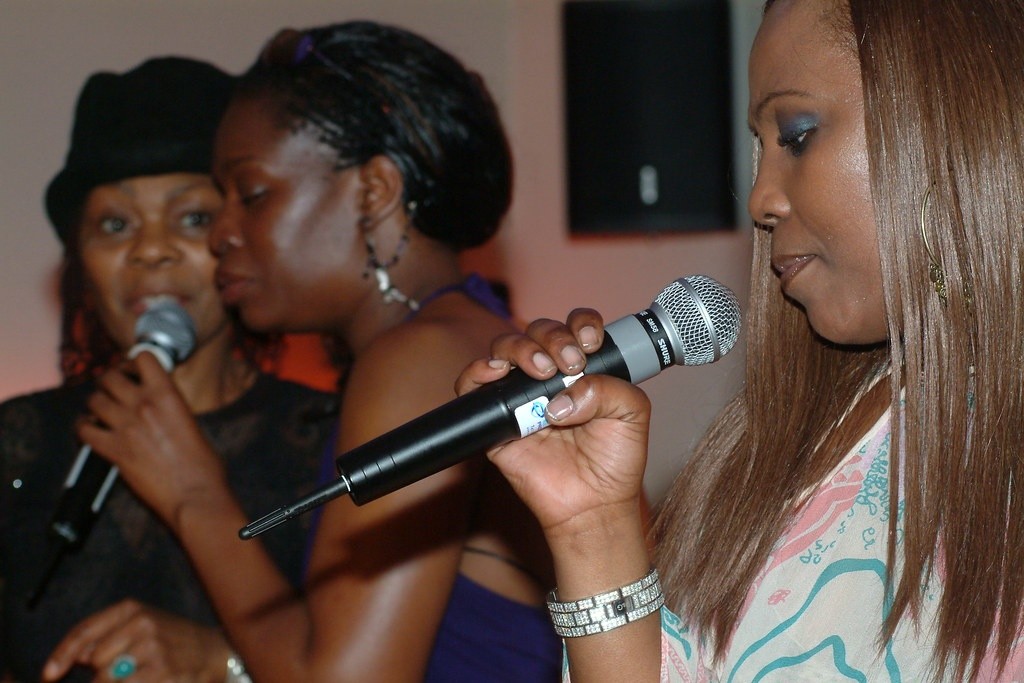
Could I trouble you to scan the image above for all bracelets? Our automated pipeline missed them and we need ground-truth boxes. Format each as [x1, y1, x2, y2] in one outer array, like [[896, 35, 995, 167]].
[[546, 568, 665, 637]]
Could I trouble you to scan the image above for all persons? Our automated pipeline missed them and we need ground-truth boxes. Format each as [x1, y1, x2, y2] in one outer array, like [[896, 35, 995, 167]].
[[1, 0, 1024, 683]]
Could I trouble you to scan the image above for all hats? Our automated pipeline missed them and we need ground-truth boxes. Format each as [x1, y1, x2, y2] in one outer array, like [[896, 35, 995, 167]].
[[44, 56, 231, 241]]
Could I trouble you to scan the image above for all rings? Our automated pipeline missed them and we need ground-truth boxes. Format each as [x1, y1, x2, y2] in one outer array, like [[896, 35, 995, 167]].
[[109, 655, 135, 680]]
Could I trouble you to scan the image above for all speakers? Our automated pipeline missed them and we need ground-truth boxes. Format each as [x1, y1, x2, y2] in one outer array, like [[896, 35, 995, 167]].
[[562, 0, 737, 234]]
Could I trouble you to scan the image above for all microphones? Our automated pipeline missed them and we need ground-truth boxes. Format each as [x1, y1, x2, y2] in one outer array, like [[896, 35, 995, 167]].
[[23, 303, 201, 607], [238, 274, 741, 541]]
